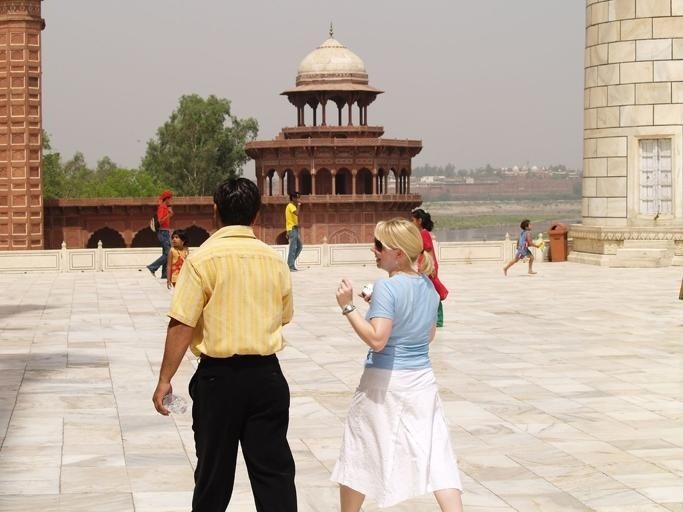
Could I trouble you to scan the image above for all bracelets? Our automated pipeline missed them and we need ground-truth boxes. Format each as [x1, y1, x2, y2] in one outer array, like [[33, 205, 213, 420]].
[[297, 202, 302, 206]]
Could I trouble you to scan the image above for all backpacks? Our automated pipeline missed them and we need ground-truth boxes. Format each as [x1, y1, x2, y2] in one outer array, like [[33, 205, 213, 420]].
[[150, 216, 157, 231]]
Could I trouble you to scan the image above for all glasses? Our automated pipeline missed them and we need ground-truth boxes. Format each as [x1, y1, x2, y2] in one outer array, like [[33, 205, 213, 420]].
[[375, 239, 384, 251]]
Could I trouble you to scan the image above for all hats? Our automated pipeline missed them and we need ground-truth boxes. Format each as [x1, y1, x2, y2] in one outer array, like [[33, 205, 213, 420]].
[[160, 192, 171, 200]]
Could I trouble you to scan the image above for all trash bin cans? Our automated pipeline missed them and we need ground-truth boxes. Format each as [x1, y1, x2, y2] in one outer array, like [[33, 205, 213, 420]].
[[548, 223, 567, 261]]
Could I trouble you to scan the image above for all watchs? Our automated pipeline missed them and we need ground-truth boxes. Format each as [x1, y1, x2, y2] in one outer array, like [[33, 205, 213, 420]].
[[343, 305, 356, 315]]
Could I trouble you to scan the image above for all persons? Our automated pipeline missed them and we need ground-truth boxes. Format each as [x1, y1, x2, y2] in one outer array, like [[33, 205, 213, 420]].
[[153, 177, 297, 512], [285, 191, 303, 271], [332, 217, 463, 512], [167, 230, 189, 289], [146, 191, 174, 279], [503, 220, 540, 276], [409, 209, 449, 327]]
